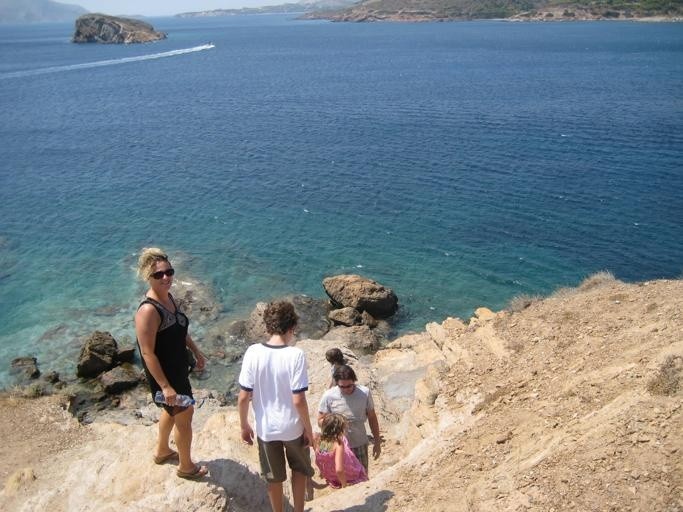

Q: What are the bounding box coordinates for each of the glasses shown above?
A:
[149,268,174,280]
[337,383,353,388]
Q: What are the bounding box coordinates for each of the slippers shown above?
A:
[177,464,208,480]
[153,450,179,464]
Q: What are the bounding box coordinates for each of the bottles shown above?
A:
[154,389,196,408]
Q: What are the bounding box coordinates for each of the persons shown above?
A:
[135,248,208,481]
[309,412,370,489]
[237,300,317,511]
[316,365,381,478]
[324,348,347,389]
